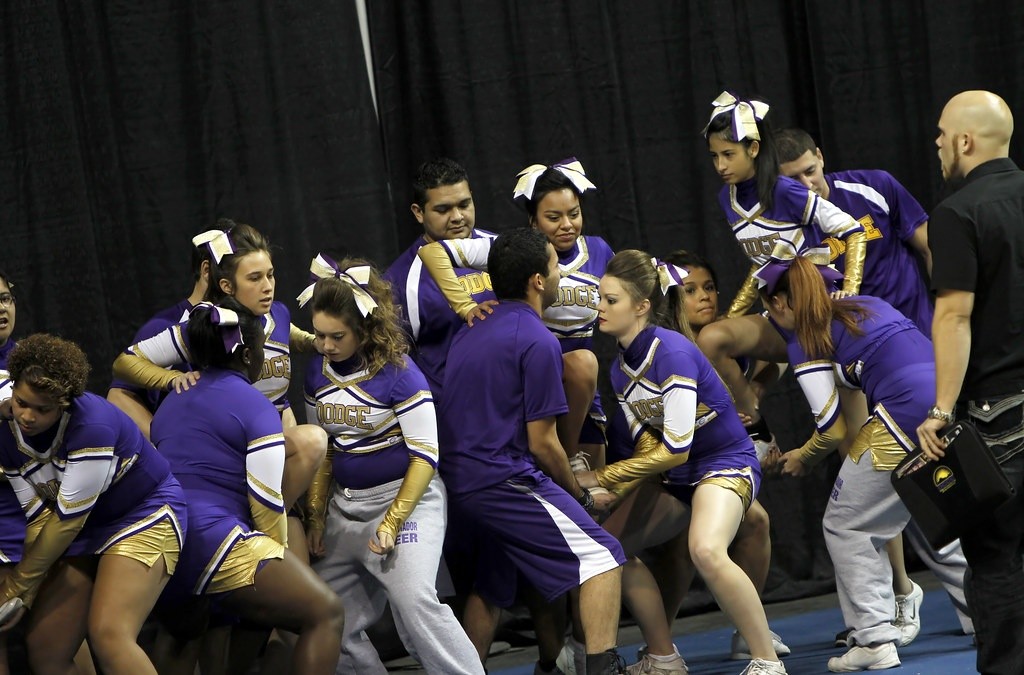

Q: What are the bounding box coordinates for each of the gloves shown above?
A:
[0,569,44,610]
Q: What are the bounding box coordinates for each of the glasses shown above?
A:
[0,293,15,307]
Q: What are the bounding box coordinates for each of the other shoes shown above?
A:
[533,660,566,675]
[585,649,628,675]
[0,597,27,631]
[555,645,578,675]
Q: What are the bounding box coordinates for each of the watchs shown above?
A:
[927,404,953,425]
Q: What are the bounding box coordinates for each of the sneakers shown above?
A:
[624,642,689,675]
[748,431,781,469]
[742,659,788,675]
[893,579,923,646]
[828,641,901,672]
[730,627,791,659]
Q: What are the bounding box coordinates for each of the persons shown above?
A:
[915,90,1024,675]
[0,93,978,675]
